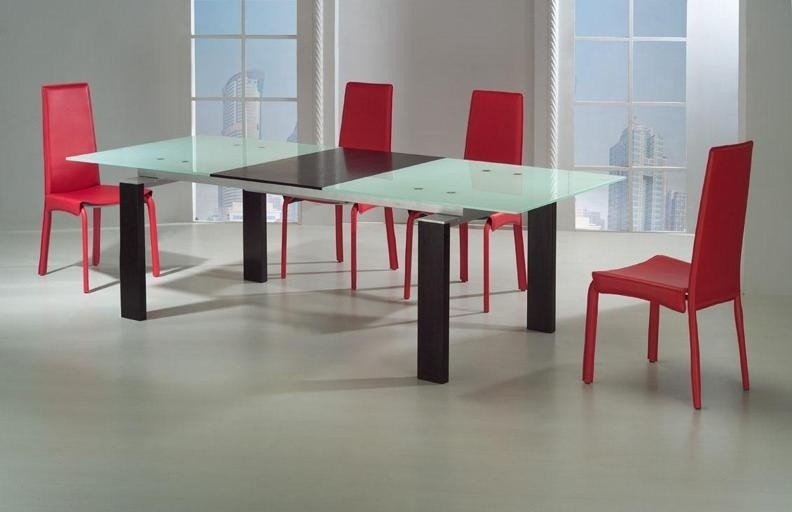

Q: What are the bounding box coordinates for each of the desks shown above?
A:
[65,134,626,384]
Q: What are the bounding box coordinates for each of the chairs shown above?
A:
[37,83,161,294]
[581,141,751,410]
